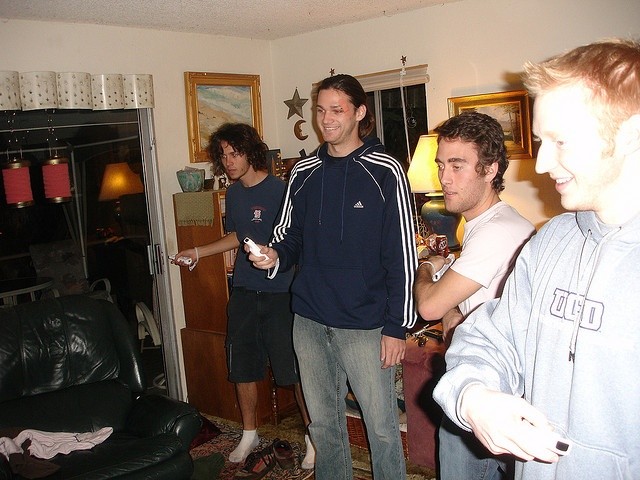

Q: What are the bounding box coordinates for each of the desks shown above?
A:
[0,274,58,306]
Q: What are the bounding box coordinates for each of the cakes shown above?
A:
[417,244,429,260]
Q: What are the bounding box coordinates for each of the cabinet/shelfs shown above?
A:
[176,328,298,427]
[174,191,239,333]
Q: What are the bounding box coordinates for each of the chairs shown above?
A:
[29,238,113,304]
[134,276,163,355]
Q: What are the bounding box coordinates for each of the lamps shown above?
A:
[42,112,72,205]
[98,159,144,234]
[0,111,36,210]
[406,134,462,249]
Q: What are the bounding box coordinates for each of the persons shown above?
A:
[412,111,537,479]
[244,75,420,480]
[171,123,317,469]
[431,40,640,479]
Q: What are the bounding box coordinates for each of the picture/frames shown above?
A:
[446,89,534,161]
[184,71,264,163]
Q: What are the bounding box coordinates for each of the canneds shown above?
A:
[437,236,448,257]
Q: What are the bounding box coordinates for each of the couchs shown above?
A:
[0,292,225,480]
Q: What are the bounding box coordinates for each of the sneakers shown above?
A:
[232,446,276,479]
[272,438,295,470]
